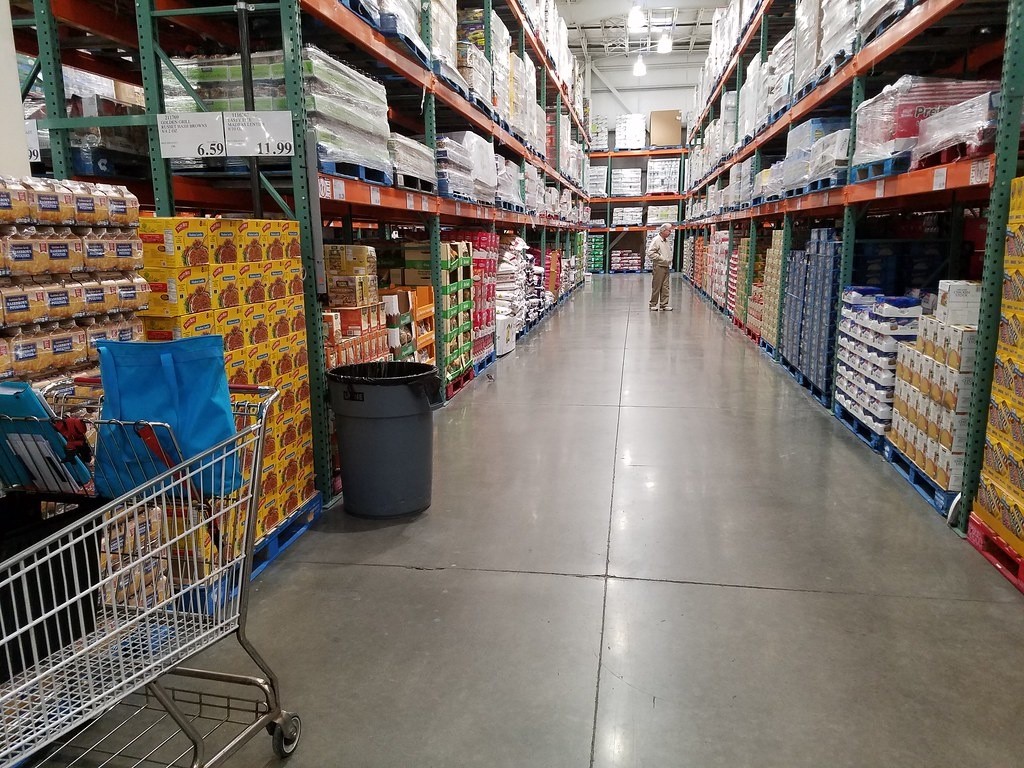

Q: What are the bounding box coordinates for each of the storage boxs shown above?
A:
[9,1,593,587]
[682,0,996,219]
[683,223,980,493]
[587,205,678,271]
[974,176,1024,558]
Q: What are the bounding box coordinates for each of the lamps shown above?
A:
[657,32,672,54]
[633,53,646,76]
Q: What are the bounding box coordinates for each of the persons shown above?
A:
[648,223,673,312]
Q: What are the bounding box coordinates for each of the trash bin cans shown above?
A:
[322,352,457,535]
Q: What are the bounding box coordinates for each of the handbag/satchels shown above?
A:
[96,334,246,502]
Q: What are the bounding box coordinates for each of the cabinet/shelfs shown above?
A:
[587,147,690,274]
[679,0,1024,594]
[0,0,594,648]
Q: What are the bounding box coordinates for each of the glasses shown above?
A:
[665,230,671,232]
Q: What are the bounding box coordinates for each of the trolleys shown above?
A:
[1,375,303,768]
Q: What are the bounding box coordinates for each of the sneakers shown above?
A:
[648,307,658,311]
[660,307,673,310]
